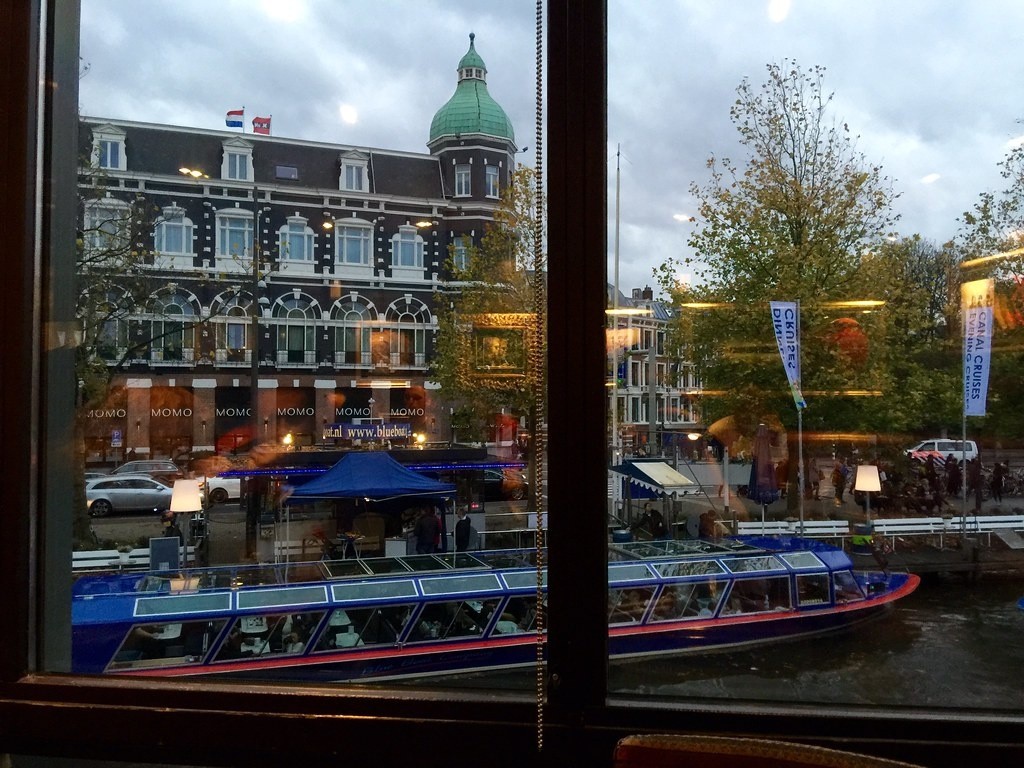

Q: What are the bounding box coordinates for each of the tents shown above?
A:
[284,452,457,571]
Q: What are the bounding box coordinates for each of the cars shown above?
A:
[84,471,172,519]
[193,472,240,505]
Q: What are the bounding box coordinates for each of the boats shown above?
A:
[72,534,922,686]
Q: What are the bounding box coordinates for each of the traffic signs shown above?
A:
[111,429,123,448]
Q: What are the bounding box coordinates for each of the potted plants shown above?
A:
[940,513,953,527]
[784,517,799,530]
[117,545,133,562]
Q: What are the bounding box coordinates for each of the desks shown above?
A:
[329,610,351,626]
[496,621,525,633]
[465,600,483,613]
[241,615,268,633]
[335,633,365,647]
[241,637,270,654]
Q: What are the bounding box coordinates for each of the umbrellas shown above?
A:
[746,423,779,537]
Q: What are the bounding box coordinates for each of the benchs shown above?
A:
[738,521,853,550]
[70,545,196,573]
[873,515,1024,547]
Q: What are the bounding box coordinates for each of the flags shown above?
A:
[252,117,270,134]
[226,110,243,128]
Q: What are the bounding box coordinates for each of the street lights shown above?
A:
[368,398,376,452]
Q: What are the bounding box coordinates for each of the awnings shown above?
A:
[611,461,700,495]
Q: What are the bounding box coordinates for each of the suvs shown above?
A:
[903,439,978,472]
[110,459,189,489]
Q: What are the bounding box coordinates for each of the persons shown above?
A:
[455,508,472,552]
[271,613,306,654]
[776,453,1010,503]
[626,502,664,541]
[132,624,163,637]
[219,626,255,660]
[412,502,442,554]
[698,510,716,538]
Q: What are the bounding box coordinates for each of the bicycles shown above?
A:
[939,463,1024,502]
